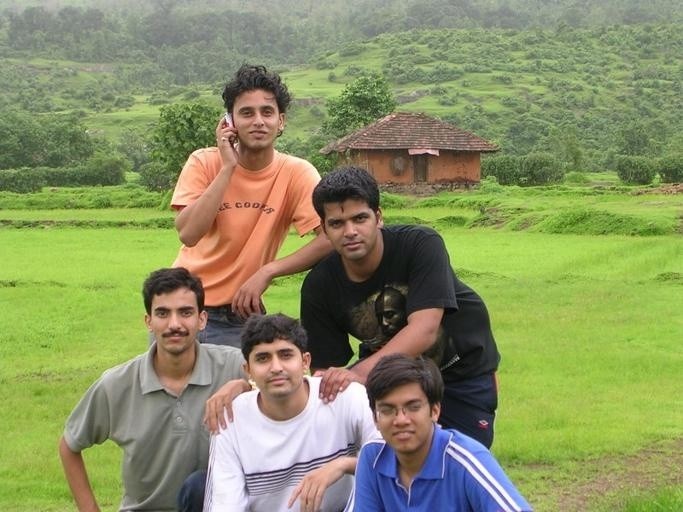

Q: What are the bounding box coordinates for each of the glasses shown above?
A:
[374,400,428,418]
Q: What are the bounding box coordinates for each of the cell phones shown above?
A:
[223,112,237,143]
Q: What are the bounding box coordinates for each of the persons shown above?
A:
[202,313,386,512]
[343,352,534,512]
[300,167,501,450]
[58,266,254,512]
[149,62,335,348]
[359,288,456,370]
